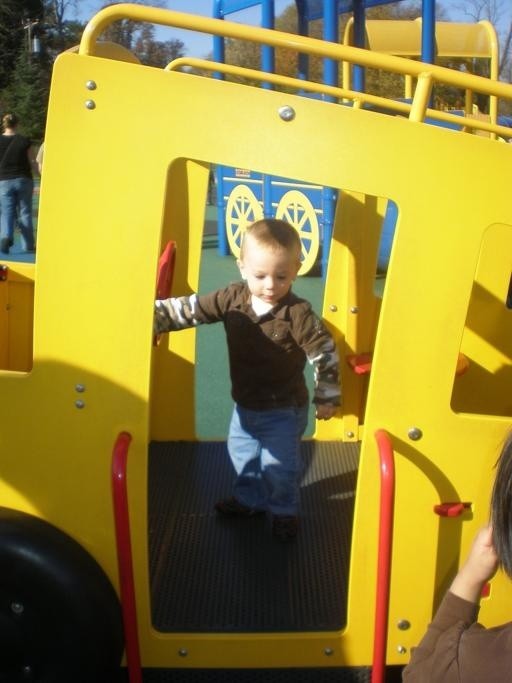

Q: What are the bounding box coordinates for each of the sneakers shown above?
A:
[269,514,300,544]
[213,498,266,517]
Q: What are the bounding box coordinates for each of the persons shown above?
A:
[36,142,45,173]
[155,218,343,543]
[396,429,510,683]
[1,112,36,252]
